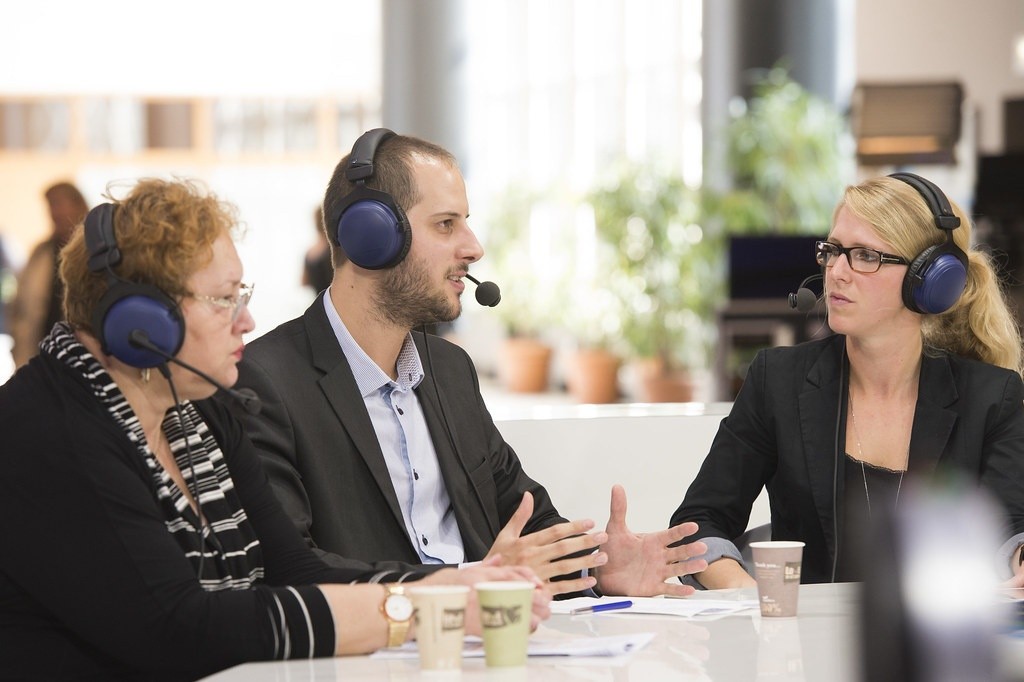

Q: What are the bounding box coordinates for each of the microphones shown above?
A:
[787,274,823,313]
[465,274,502,307]
[156,350,263,418]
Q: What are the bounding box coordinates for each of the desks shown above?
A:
[202,578,866,682]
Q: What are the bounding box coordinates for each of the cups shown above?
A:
[411,585,471,678]
[473,580,536,667]
[748,540,806,618]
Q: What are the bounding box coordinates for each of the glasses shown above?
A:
[814,241,911,273]
[182,284,254,323]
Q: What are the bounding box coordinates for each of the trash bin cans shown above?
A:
[570,601,633,615]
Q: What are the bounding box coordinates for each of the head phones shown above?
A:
[886,172,971,314]
[82,202,187,379]
[334,128,413,271]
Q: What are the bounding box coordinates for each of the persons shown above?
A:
[9,183,91,370]
[663,172,1024,682]
[230,127,710,599]
[0,176,548,682]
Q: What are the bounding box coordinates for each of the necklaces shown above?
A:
[848,389,910,520]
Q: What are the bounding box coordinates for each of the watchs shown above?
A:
[378,580,415,648]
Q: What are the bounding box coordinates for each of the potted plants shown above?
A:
[492,169,721,403]
